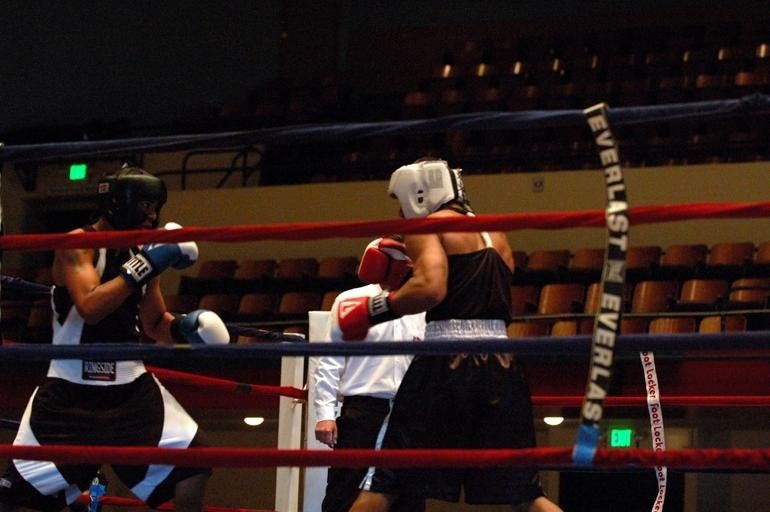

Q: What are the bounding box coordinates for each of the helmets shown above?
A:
[94,162,167,230]
[388,160,470,219]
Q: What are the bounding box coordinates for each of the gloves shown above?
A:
[119,221,200,287]
[170,310,231,347]
[326,282,402,342]
[357,238,412,292]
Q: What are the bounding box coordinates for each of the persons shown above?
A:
[313,235,430,512]
[328,156,566,510]
[0,167,230,512]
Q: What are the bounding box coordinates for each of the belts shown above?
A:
[340,395,400,413]
